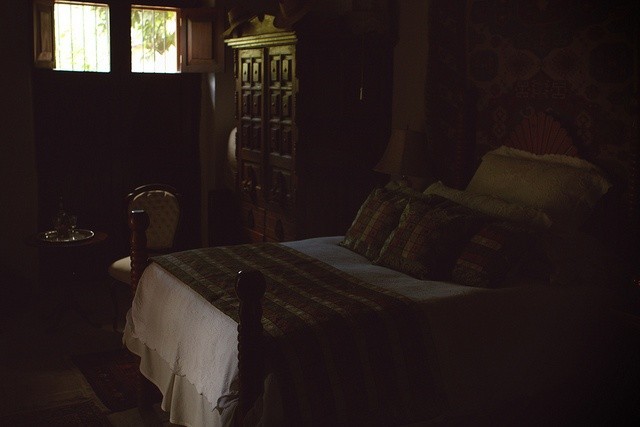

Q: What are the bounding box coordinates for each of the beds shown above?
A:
[120,208,585,426]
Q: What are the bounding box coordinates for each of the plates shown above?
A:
[38,229,95,243]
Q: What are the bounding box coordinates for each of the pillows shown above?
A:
[422,180,550,229]
[374,194,529,289]
[464,146,610,221]
[336,185,407,265]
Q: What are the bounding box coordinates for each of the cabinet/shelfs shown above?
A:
[224,31,310,243]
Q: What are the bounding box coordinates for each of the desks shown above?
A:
[25,224,110,336]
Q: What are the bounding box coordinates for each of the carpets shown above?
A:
[64,347,142,416]
[0,399,116,427]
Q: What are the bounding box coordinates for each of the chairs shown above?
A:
[108,182,185,336]
